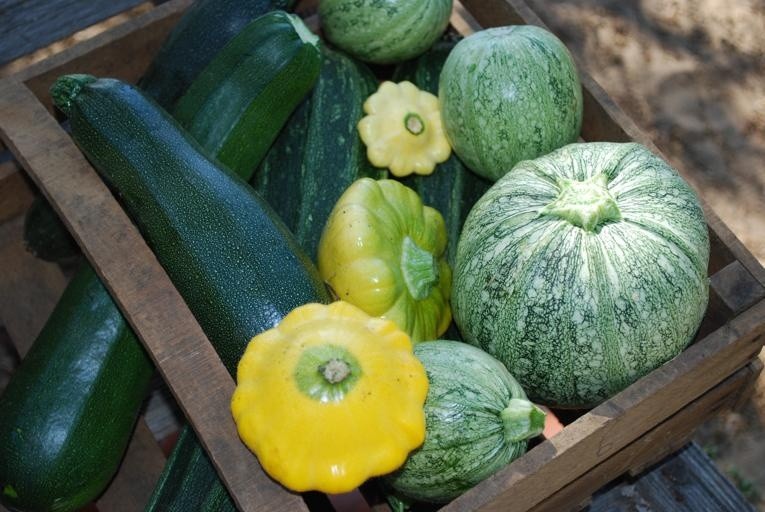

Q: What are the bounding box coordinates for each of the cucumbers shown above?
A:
[0,0,494,512]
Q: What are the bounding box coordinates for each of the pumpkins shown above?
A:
[227,0,712,498]
